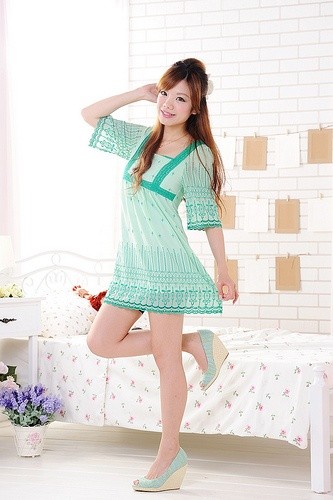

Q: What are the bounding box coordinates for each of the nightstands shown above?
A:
[0,292,40,382]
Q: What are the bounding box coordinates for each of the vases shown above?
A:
[1,428,54,467]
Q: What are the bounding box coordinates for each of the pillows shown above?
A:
[40,284,150,337]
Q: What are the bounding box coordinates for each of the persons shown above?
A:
[81,57,239,492]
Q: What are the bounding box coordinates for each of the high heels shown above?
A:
[133,447,187,491]
[197,329,228,391]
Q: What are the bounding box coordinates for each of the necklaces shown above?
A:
[158,132,188,148]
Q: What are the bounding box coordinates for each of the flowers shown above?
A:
[1,383,64,426]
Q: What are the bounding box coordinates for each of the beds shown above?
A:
[1,250,333,489]
